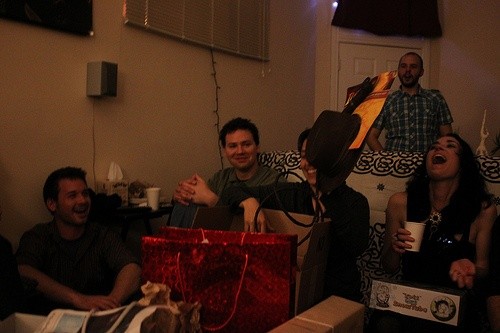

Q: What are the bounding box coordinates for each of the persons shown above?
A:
[166,115,287,230]
[219,126,371,303]
[368,49,454,151]
[368,131,500,332]
[14,165,144,313]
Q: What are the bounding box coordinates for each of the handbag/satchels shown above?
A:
[255,171,333,315]
[138,226,298,333]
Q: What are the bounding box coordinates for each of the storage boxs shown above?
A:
[191,207,331,315]
[266,294,365,333]
[369,281,467,326]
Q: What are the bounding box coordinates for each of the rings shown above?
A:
[390,232,400,241]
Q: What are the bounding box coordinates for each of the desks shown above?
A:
[98,204,171,240]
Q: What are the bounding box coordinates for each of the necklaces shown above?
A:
[429,206,444,241]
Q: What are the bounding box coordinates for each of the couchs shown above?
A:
[251,152,500,322]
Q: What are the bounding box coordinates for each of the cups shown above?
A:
[404,221,425,252]
[146,188,161,212]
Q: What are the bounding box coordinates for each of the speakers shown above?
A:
[86,60,118,98]
